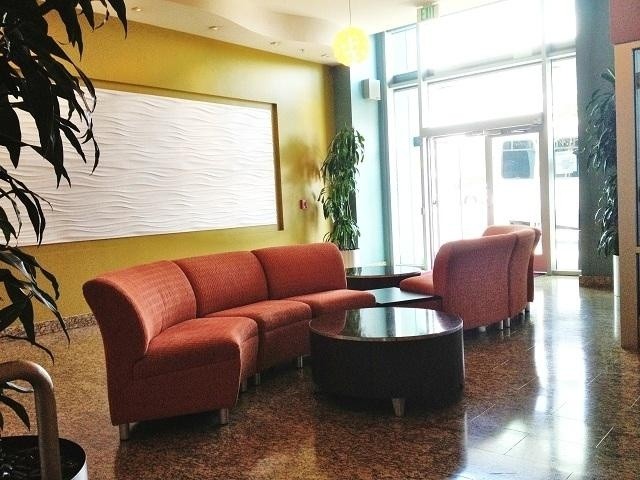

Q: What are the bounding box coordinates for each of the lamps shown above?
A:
[332,0,369,68]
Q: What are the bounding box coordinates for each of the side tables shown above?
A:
[366,286,442,311]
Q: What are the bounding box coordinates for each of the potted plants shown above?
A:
[316,118,366,268]
[0,0,127,480]
[583,67,621,296]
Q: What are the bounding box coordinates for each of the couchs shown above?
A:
[82,260,259,441]
[251,243,375,318]
[172,251,313,387]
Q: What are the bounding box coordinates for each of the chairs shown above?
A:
[400,224,540,332]
